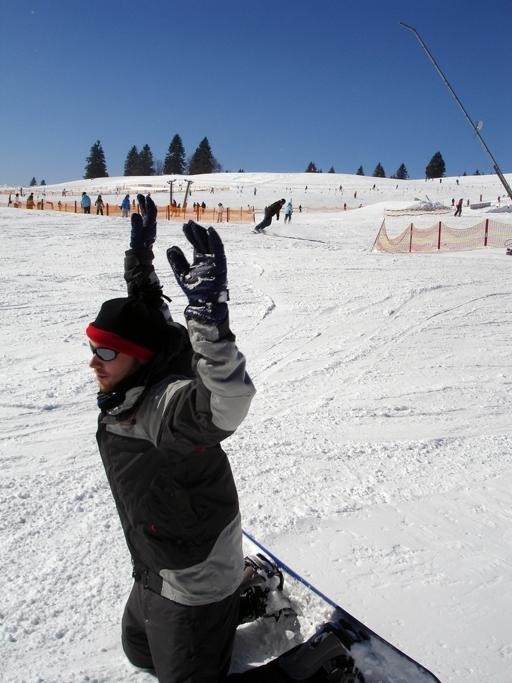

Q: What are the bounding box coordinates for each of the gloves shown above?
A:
[130,194,158,254]
[166,220,227,307]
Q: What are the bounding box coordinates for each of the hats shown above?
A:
[86,299,173,357]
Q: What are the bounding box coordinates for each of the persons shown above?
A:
[172,200,206,215]
[95,194,104,215]
[121,193,131,217]
[81,191,91,214]
[217,202,224,223]
[7,188,68,209]
[254,199,286,233]
[453,198,463,216]
[86,190,363,683]
[284,203,293,222]
[339,178,501,210]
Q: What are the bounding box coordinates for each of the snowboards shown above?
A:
[242,531,440,683]
[251,229,266,234]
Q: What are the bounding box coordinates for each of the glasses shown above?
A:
[87,338,118,361]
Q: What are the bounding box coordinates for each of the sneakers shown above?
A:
[244,553,298,624]
[308,616,372,683]
